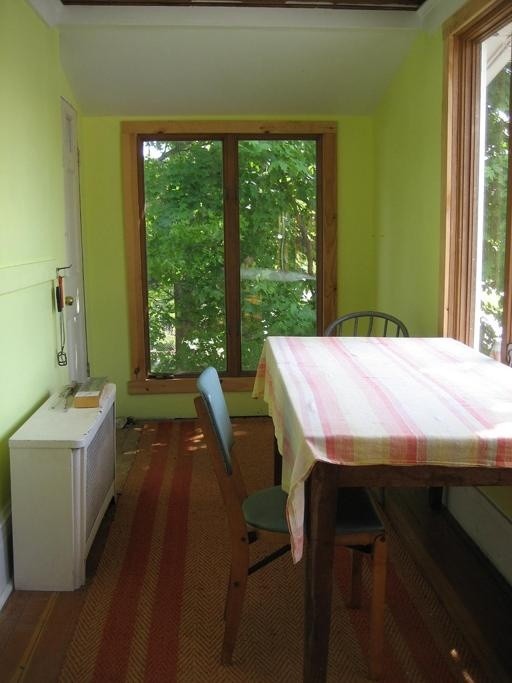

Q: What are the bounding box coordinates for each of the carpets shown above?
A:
[57,416,484,682]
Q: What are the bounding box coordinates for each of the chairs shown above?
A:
[194,367,388,683]
[323,310,409,337]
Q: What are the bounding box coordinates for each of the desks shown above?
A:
[8,382,119,592]
[251,335,512,682]
